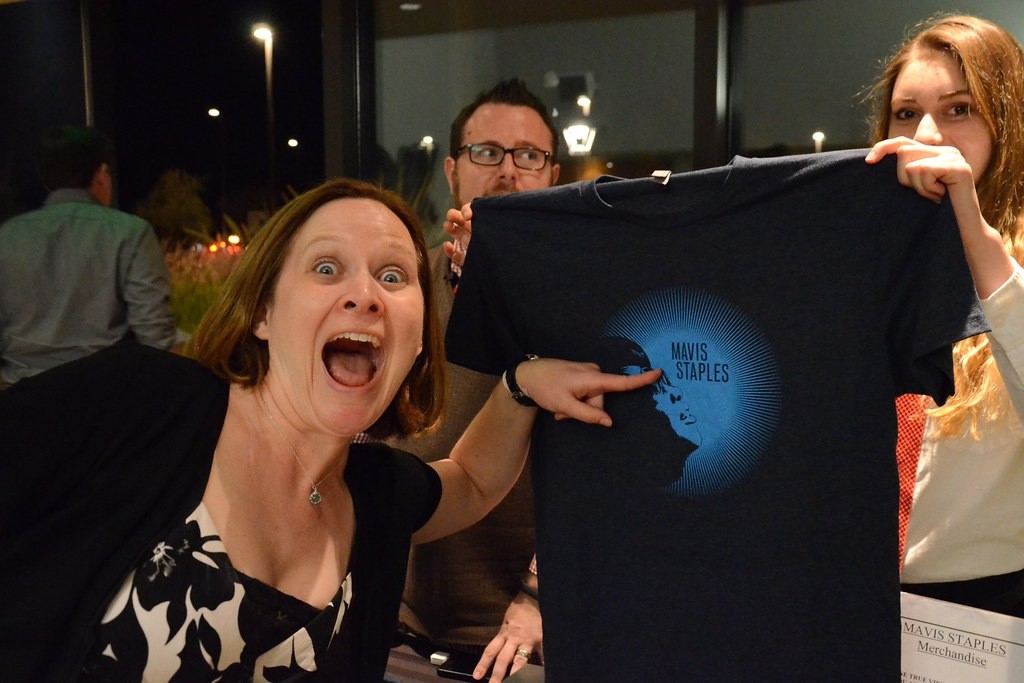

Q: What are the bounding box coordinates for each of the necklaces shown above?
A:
[259,389,342,504]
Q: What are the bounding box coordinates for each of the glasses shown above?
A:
[454,142,556,171]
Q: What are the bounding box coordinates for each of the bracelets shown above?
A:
[502,353,539,408]
[517,577,539,600]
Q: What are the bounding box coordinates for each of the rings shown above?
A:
[517,649,531,658]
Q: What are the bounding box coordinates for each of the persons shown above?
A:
[0,176,661,683]
[439,14,1024,615]
[381,75,544,683]
[0,125,181,391]
